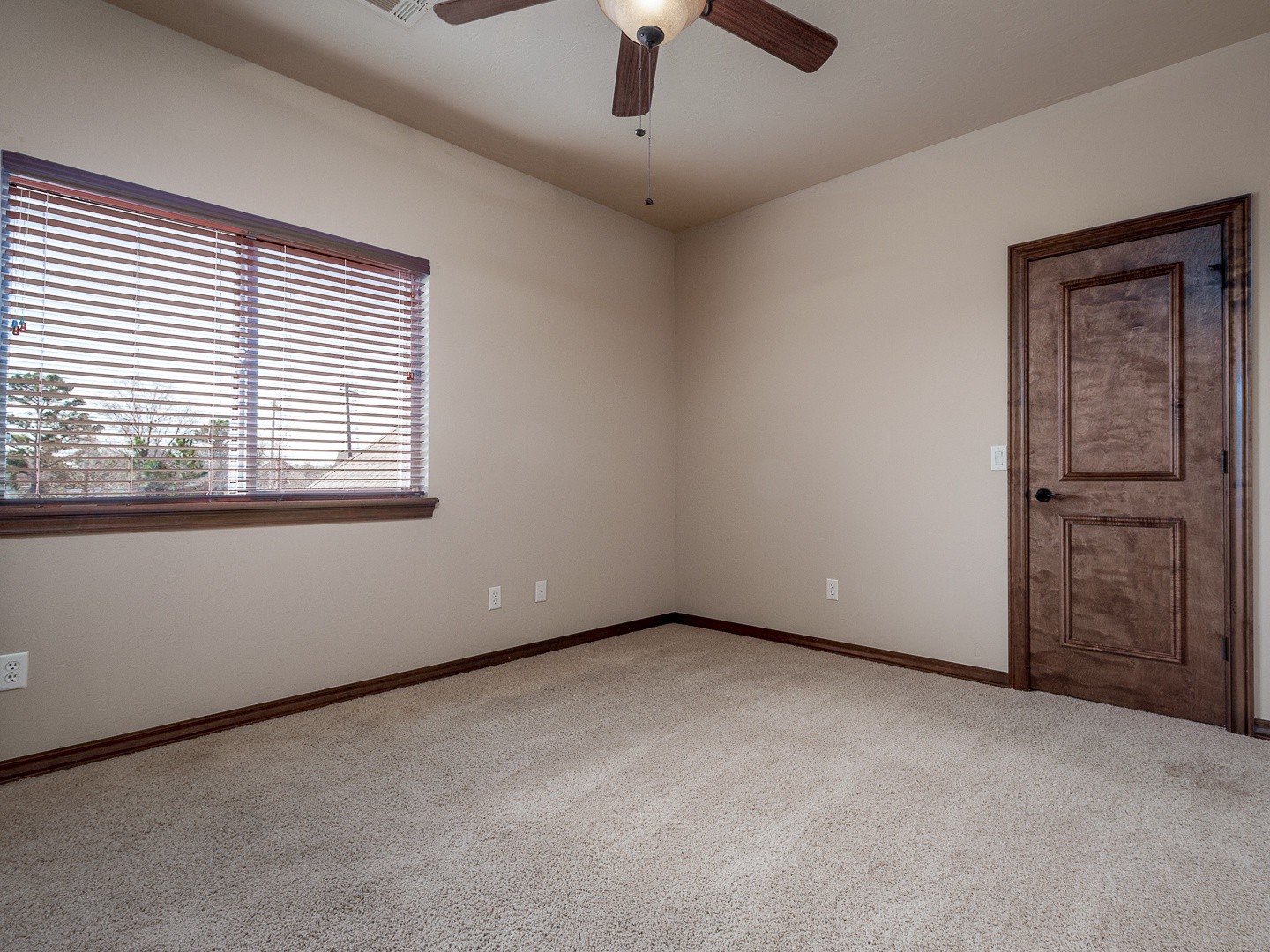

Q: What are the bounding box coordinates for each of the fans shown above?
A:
[432,0,837,116]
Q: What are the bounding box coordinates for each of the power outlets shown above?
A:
[825,579,838,600]
[0,650,29,691]
[488,587,501,610]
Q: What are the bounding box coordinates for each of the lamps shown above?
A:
[600,1,708,206]
